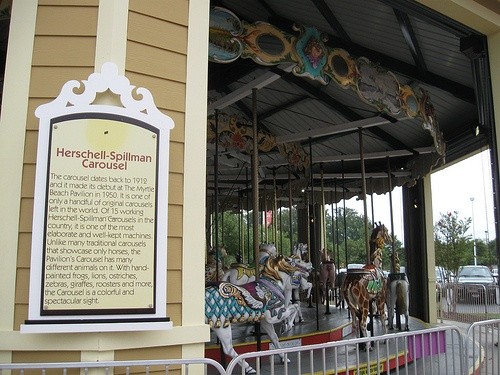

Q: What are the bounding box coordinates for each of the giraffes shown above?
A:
[343,221,392,352]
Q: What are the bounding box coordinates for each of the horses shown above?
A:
[205,243,227,281]
[204,255,301,374]
[384,248,410,332]
[222,242,277,285]
[284,242,314,327]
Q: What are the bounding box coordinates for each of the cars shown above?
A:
[434,265,499,305]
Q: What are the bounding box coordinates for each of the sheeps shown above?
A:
[319,248,336,291]
